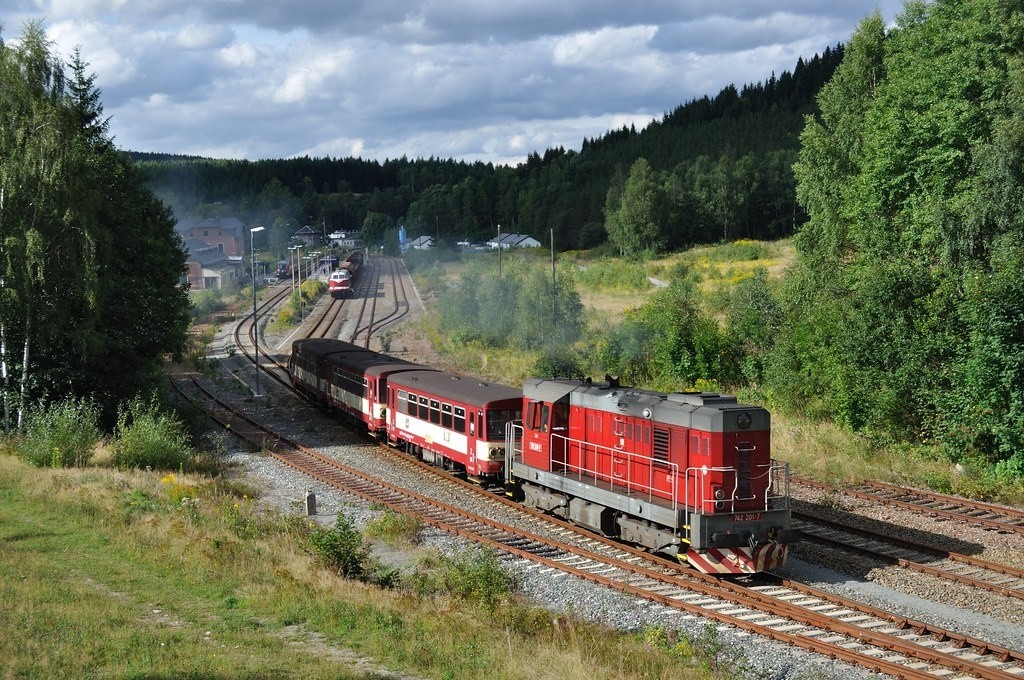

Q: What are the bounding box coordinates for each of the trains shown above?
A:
[287,338,802,575]
[328,249,366,299]
[275,253,298,279]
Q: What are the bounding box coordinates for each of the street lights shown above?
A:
[251,226,265,396]
